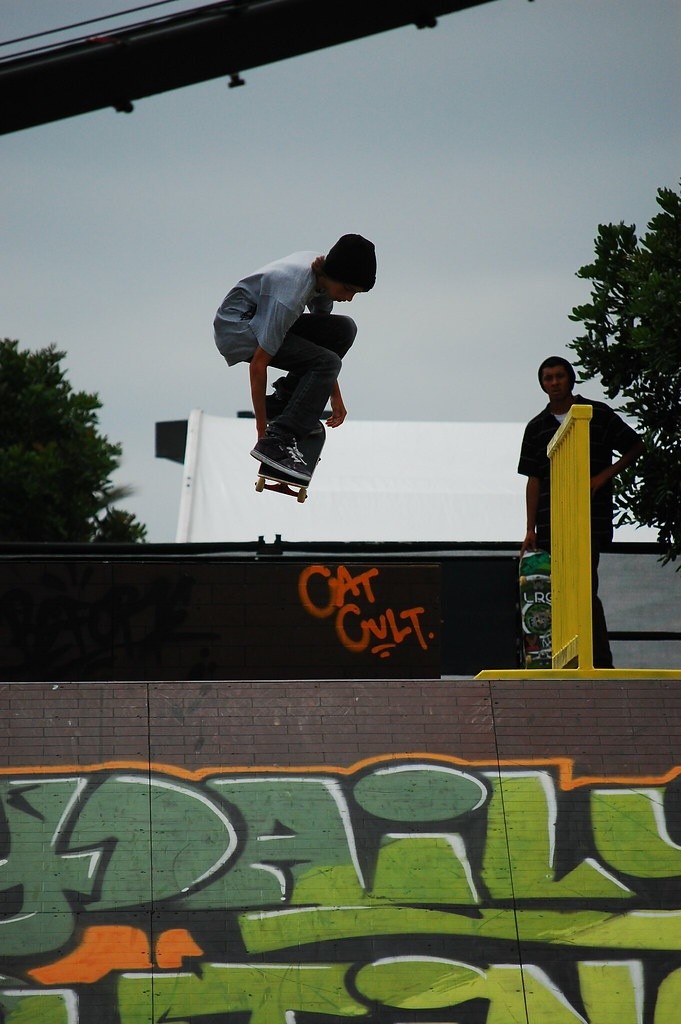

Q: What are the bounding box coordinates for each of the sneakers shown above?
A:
[265,396,323,435]
[251,436,311,482]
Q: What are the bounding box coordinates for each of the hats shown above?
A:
[323,234,377,290]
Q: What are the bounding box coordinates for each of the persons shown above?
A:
[213,234,377,480]
[518,356,646,669]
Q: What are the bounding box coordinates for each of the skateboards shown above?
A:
[518,548,551,668]
[254,417,326,503]
